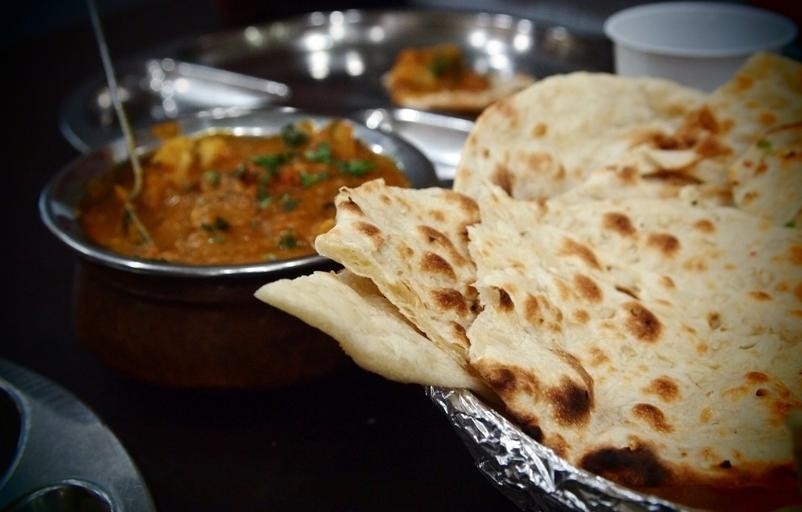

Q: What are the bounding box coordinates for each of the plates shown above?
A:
[39,110,437,303]
[194,5,605,120]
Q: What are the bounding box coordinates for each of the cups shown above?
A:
[604,4,797,94]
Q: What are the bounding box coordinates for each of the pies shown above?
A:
[254,50,802,512]
